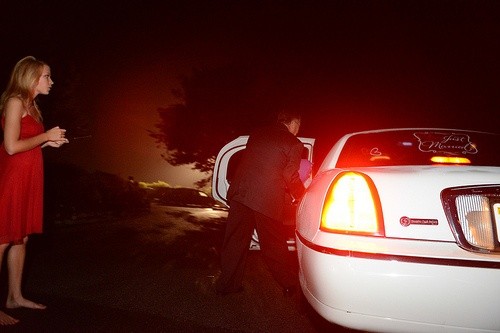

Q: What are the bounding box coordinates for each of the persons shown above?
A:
[211,107,305,297]
[0,55,69,328]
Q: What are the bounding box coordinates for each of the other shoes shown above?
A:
[284,285,297,297]
[216,286,244,297]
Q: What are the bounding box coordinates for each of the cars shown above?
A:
[212,127,500,333]
